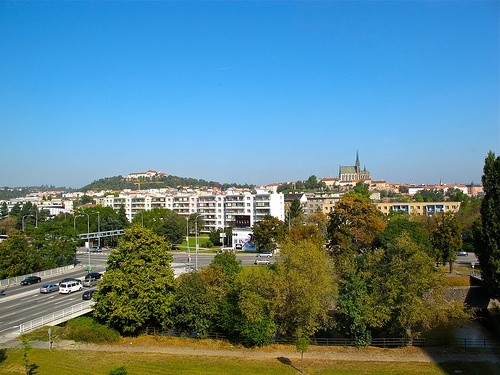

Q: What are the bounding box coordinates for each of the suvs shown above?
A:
[256,252,273,258]
[90,246,102,252]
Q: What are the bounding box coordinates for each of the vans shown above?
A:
[58,281,83,294]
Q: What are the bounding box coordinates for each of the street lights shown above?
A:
[73,214,84,230]
[97,211,100,246]
[78,211,99,274]
[22,214,33,232]
[195,213,206,272]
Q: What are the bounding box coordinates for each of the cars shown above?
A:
[82,290,98,300]
[59,278,82,285]
[457,249,468,257]
[255,258,271,265]
[20,276,41,286]
[85,272,103,279]
[83,278,100,288]
[40,284,59,293]
